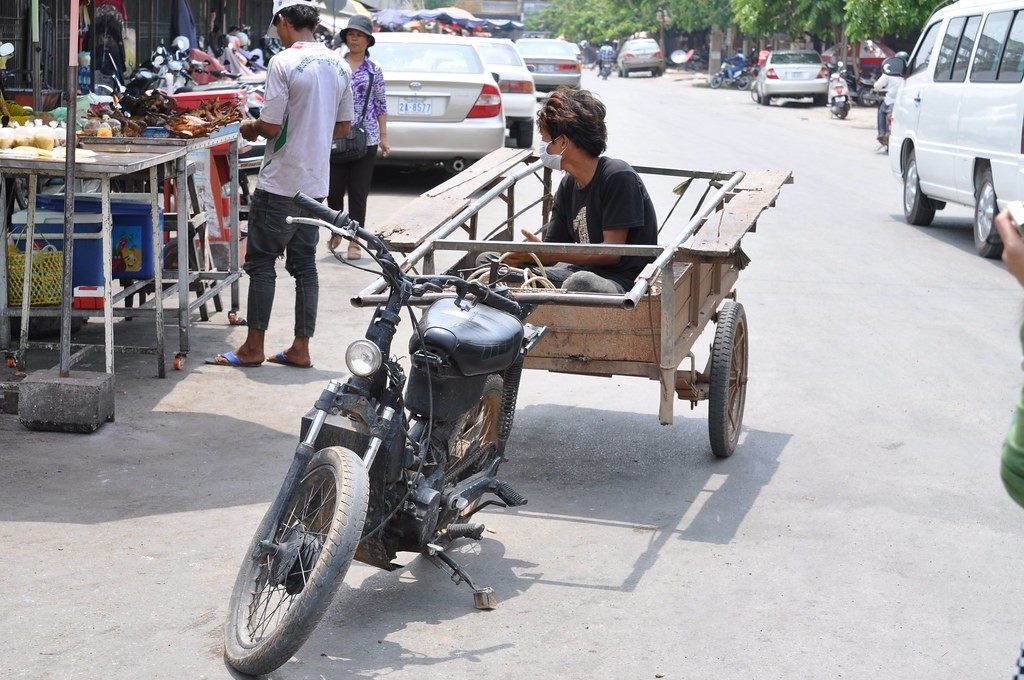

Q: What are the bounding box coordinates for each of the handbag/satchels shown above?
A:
[329,122,366,163]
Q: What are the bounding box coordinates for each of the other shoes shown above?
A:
[333,236,341,245]
[347,244,362,259]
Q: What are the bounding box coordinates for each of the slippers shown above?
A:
[266,352,313,368]
[205,350,261,367]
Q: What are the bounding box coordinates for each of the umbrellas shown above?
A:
[819,39,897,65]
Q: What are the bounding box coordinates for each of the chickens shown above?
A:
[82,91,242,141]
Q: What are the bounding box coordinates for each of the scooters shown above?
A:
[111,25,259,122]
[225,192,548,674]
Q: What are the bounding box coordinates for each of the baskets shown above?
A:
[6,224,62,306]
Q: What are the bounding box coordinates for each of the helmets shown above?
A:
[171,36,189,52]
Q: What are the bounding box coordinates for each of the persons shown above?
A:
[994,208,1024,680]
[471,86,657,294]
[202,1,357,369]
[872,50,909,136]
[226,24,252,65]
[326,15,392,261]
[597,38,615,74]
[725,48,747,82]
[759,45,775,69]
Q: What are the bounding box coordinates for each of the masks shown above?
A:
[539,136,570,172]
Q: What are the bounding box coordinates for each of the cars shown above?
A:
[752,48,833,108]
[620,38,664,77]
[332,32,536,182]
[514,37,581,101]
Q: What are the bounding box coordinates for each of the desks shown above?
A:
[5,89,62,113]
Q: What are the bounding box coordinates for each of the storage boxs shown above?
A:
[35,193,165,281]
[10,209,104,296]
[75,287,105,310]
[168,90,247,150]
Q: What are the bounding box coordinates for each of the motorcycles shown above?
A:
[870,88,896,153]
[827,63,853,118]
[710,59,750,90]
[847,75,886,106]
[594,61,616,80]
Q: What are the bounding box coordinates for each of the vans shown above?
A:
[888,0,1024,259]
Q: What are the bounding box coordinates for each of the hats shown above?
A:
[340,14,375,47]
[266,0,317,38]
